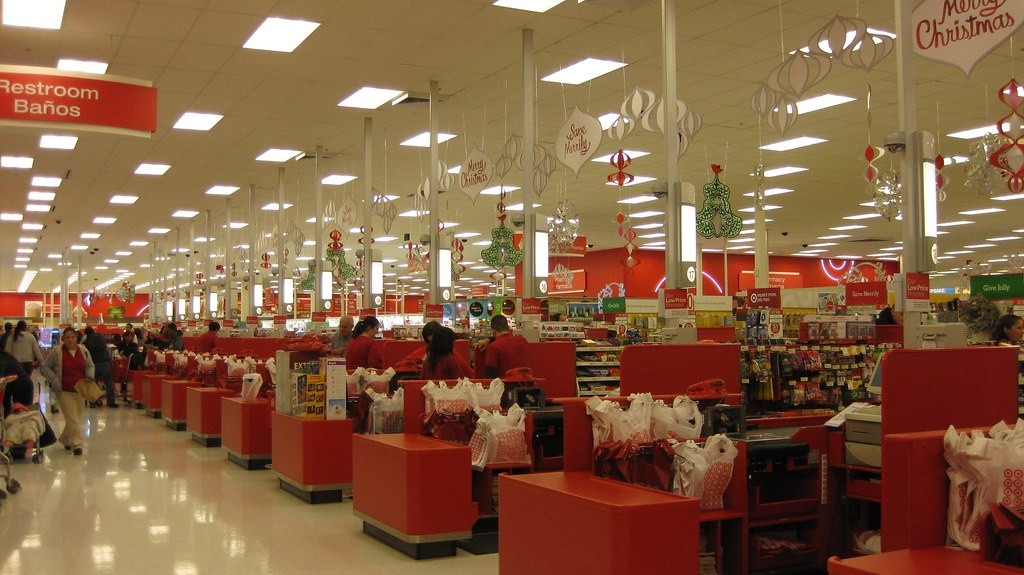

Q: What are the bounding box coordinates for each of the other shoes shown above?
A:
[109,403,120,409]
[74,448,82,456]
[58,438,71,450]
[88,402,103,408]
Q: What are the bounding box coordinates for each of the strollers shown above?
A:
[0,375,22,506]
[7,381,45,464]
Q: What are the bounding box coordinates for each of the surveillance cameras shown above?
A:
[309,260,315,268]
[90,247,100,255]
[256,273,259,275]
[882,130,908,154]
[95,279,98,280]
[272,269,278,276]
[193,251,198,254]
[355,249,363,259]
[186,254,190,257]
[243,276,250,282]
[510,212,525,227]
[782,232,788,236]
[220,281,226,286]
[588,245,593,248]
[802,244,808,248]
[56,220,61,224]
[391,265,394,267]
[652,181,667,198]
[461,239,467,242]
[419,234,430,245]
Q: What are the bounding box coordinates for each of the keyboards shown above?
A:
[850,405,882,416]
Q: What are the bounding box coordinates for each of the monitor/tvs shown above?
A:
[868,352,887,394]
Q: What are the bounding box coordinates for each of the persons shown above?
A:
[552,313,566,321]
[30,327,39,343]
[476,314,529,378]
[198,321,221,353]
[41,326,96,455]
[45,379,59,414]
[853,305,903,362]
[331,315,354,355]
[420,327,465,379]
[78,327,119,408]
[5,320,43,376]
[406,321,475,379]
[345,315,382,369]
[114,322,184,369]
[0,323,13,353]
[993,314,1024,345]
[3,402,45,456]
[0,352,34,406]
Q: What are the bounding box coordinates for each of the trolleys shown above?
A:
[94,353,134,406]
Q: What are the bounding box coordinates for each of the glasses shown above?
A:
[338,324,353,329]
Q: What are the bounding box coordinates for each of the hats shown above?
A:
[149,328,159,336]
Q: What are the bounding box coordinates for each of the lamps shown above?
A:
[165,230,454,323]
[676,200,697,290]
[531,213,549,299]
[872,162,902,222]
[653,179,667,198]
[912,129,940,273]
[546,167,581,254]
[883,129,906,152]
[511,211,526,227]
[962,77,1011,198]
